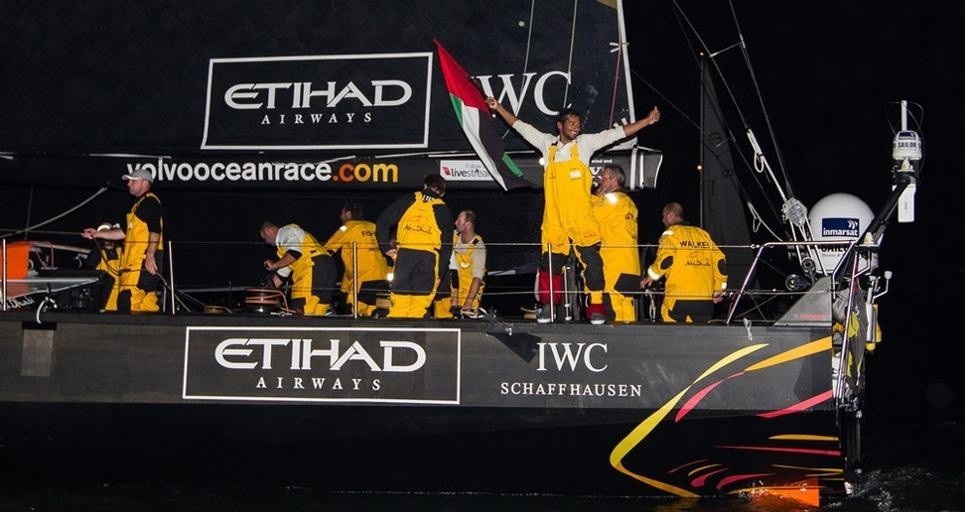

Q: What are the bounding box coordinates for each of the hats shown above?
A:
[121,169,154,183]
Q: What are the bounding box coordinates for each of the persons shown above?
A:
[484,96,663,327]
[374,174,455,321]
[259,217,339,318]
[640,202,730,325]
[589,163,643,323]
[447,211,489,319]
[79,168,168,314]
[319,200,394,320]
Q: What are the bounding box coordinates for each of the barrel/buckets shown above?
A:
[245,287,281,315]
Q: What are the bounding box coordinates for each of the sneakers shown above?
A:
[590,312,605,325]
[522,302,544,311]
[538,304,557,323]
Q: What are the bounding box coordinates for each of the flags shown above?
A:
[433,34,527,194]
